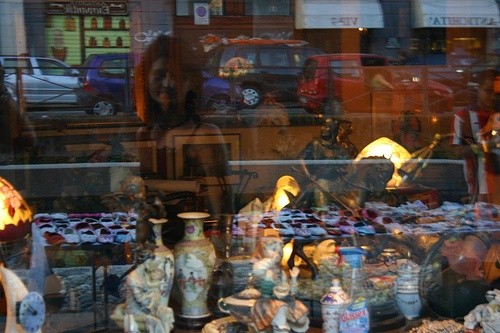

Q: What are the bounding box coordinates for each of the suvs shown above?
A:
[3,54,79,108]
[76,52,246,117]
[206,44,326,108]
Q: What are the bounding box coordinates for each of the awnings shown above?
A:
[295,1,384,32]
[410,1,500,32]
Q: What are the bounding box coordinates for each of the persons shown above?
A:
[450,71,500,208]
[0,65,38,191]
[370,69,395,90]
[337,119,357,161]
[122,35,230,248]
[393,109,422,148]
[298,116,351,223]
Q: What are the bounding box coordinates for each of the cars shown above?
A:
[297,51,500,116]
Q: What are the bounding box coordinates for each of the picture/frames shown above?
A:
[64,137,158,175]
[360,0,447,66]
[173,133,242,186]
